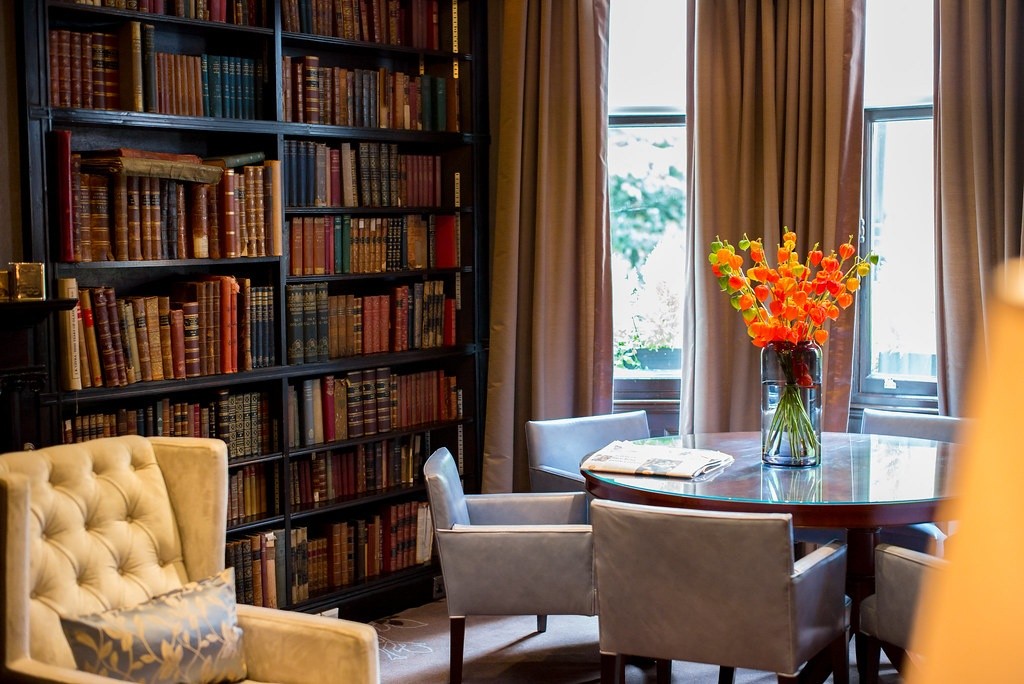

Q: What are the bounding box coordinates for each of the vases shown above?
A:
[759,340,823,470]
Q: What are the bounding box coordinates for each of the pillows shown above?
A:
[58,567,248,684]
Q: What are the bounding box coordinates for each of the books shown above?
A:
[48,0,286,611]
[282,0,466,605]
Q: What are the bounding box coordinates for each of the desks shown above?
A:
[579,432,960,684]
[0,299,78,453]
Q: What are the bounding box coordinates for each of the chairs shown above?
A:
[857,533,956,684]
[589,497,852,684]
[527,409,651,633]
[423,447,598,684]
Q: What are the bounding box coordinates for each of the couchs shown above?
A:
[0,437,381,684]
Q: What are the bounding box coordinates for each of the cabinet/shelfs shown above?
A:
[21,0,490,626]
